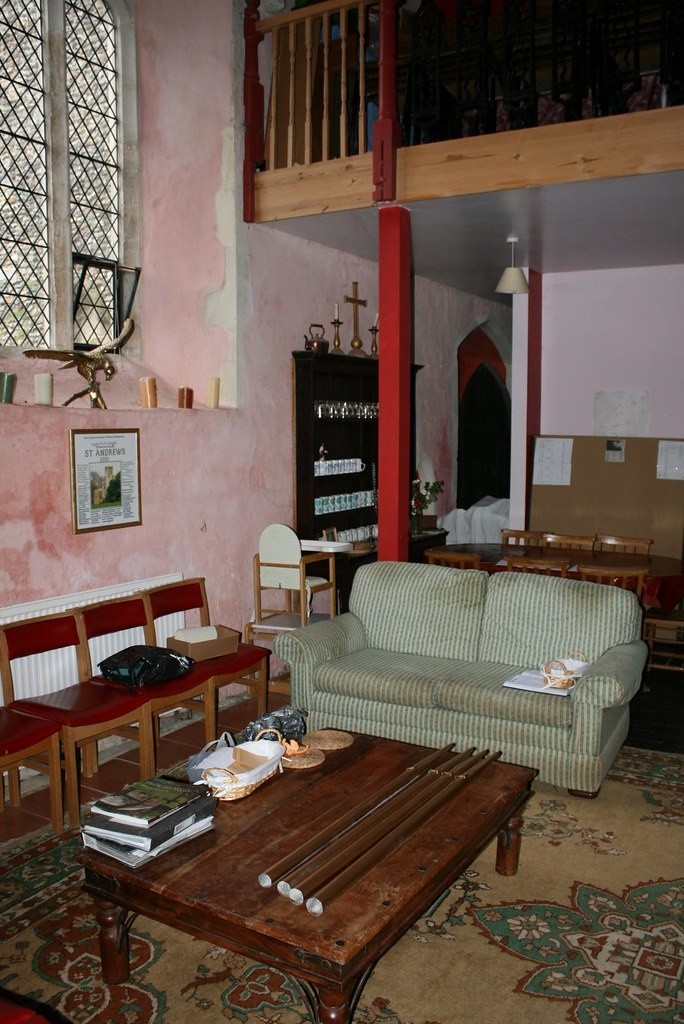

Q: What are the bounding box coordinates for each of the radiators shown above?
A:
[0,571,187,778]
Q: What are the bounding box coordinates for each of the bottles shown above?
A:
[314,400,379,418]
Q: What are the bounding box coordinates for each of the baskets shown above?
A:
[202,728,282,800]
[543,652,586,688]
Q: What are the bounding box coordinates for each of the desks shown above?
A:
[427,542,683,611]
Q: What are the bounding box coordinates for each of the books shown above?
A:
[80,777,218,868]
[501,669,571,697]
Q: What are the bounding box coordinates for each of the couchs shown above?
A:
[272,562,648,798]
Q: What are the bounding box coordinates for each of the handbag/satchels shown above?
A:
[98,645,195,692]
[188,731,234,783]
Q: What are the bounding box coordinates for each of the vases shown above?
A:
[410,509,422,536]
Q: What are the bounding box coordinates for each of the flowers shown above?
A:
[410,478,444,530]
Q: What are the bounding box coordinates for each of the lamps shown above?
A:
[494,237,531,293]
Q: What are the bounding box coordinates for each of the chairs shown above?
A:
[353,0,684,155]
[0,578,271,836]
[423,527,684,672]
[247,524,335,694]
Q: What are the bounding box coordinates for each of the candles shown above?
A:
[0,372,16,403]
[177,386,194,408]
[139,377,157,408]
[334,302,380,326]
[207,375,221,408]
[35,373,53,405]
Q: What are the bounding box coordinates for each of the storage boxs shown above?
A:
[167,624,239,660]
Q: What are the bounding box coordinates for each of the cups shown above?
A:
[139,377,157,409]
[337,525,378,542]
[34,373,53,406]
[314,490,375,515]
[0,372,17,403]
[178,386,194,408]
[314,458,366,476]
[206,378,220,409]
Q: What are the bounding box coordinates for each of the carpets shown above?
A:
[1,746,684,1024]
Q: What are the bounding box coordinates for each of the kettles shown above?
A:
[304,323,329,352]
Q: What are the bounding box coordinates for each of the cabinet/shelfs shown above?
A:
[292,350,450,615]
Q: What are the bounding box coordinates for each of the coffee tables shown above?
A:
[74,727,539,1024]
